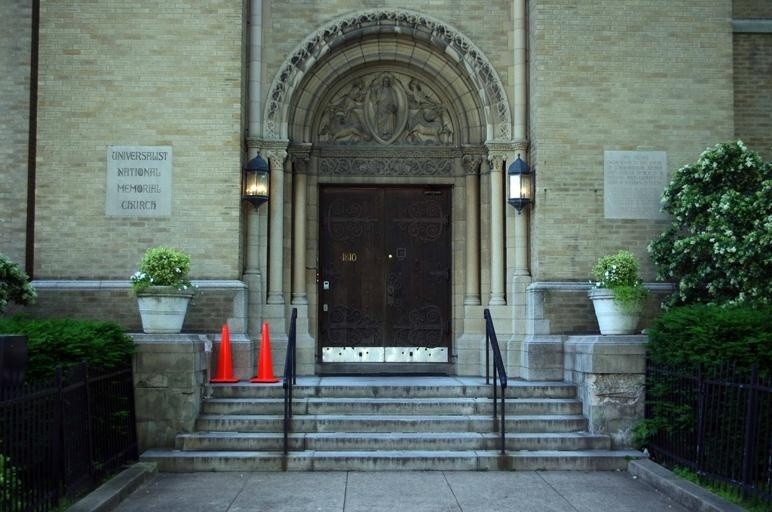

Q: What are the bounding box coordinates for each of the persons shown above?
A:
[321,73,451,146]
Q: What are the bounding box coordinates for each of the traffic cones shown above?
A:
[249,322,281,383]
[210,323,240,382]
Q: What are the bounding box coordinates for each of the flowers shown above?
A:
[127,246,199,292]
[588,245,650,314]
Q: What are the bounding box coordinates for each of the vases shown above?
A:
[590,287,644,336]
[132,285,196,336]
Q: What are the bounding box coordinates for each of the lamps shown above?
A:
[241,150,273,215]
[506,154,539,218]
